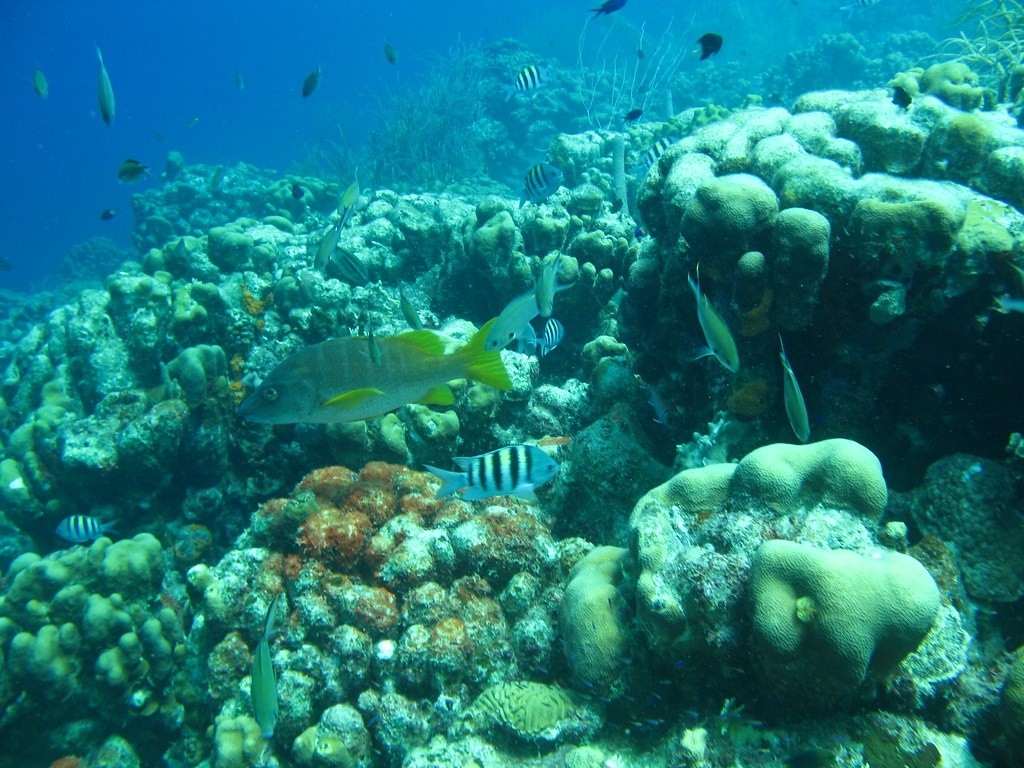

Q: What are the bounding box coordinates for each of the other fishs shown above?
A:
[2,1,1024,768]
